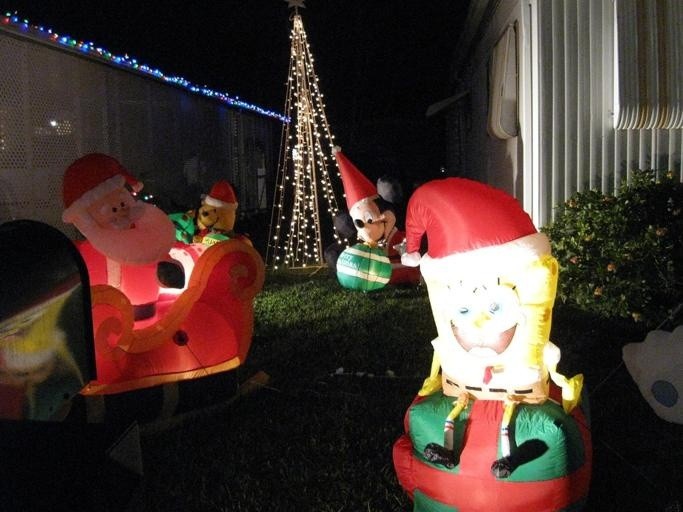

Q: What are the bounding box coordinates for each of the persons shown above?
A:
[60,151,266,398]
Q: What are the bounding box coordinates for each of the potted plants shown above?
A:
[552,168,683,429]
